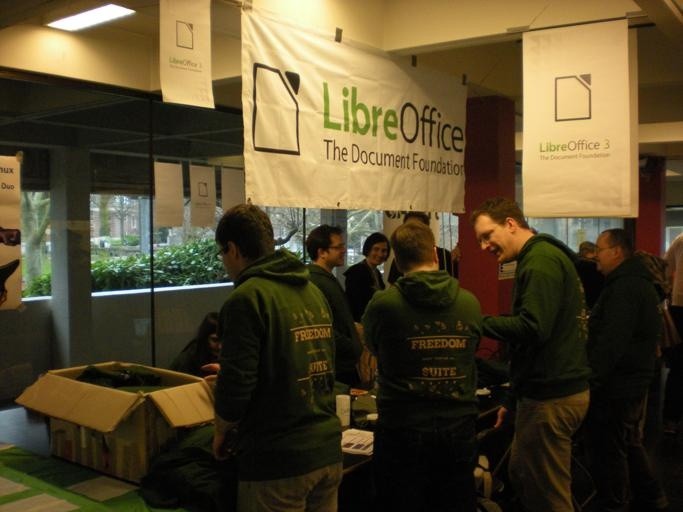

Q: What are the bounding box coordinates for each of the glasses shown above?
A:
[212,245,232,260]
[475,221,503,244]
[318,244,347,253]
[592,241,626,255]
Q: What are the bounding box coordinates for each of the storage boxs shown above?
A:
[14,358,218,482]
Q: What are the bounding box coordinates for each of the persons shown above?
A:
[307,223,360,391]
[390,212,458,287]
[589,229,666,511]
[203,204,343,511]
[344,231,389,386]
[470,196,589,511]
[663,230,683,430]
[362,224,482,511]
[572,241,599,281]
